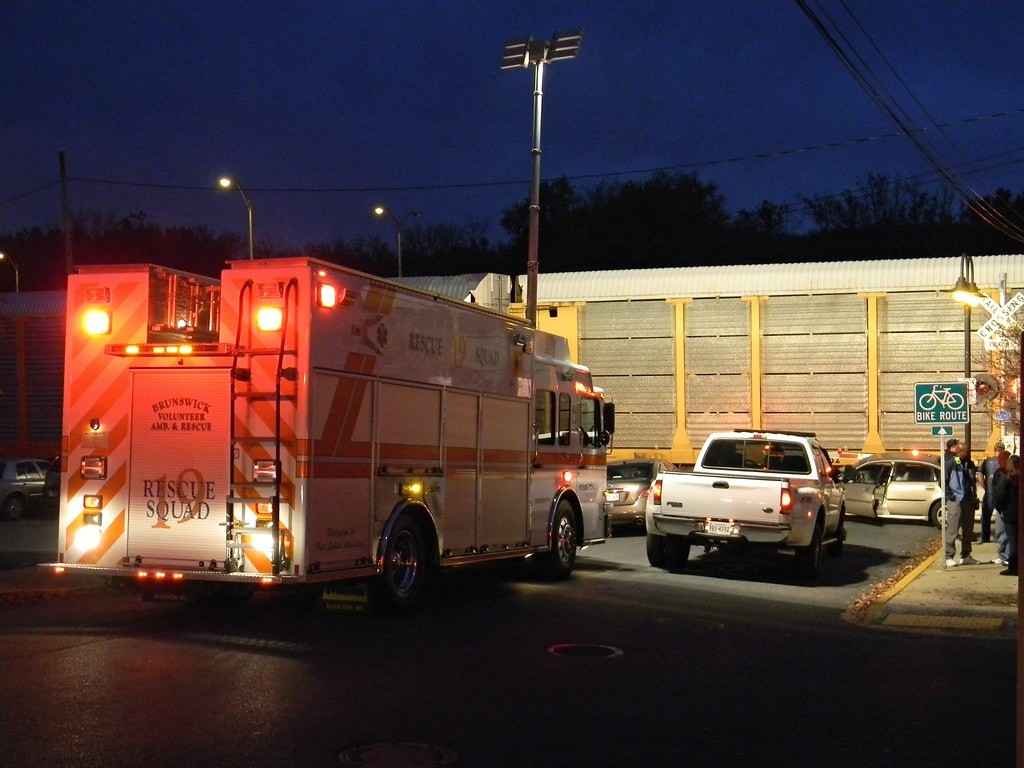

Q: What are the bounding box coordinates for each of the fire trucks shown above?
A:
[39,256,616,604]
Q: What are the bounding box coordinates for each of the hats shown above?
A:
[946,438,959,447]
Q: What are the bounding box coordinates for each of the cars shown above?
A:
[606,458,682,534]
[839,453,986,531]
[0,457,52,522]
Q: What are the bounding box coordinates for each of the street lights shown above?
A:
[220,171,282,260]
[375,199,423,276]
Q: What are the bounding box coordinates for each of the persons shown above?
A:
[999,455,1019,577]
[937,443,981,566]
[991,451,1011,564]
[976,443,1005,542]
[944,439,958,460]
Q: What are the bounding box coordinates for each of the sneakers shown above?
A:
[1002,561,1009,566]
[990,558,1002,564]
[946,559,957,566]
[960,556,980,565]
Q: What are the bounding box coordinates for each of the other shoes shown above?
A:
[1000,569,1018,576]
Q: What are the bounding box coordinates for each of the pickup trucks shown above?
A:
[645,427,846,573]
